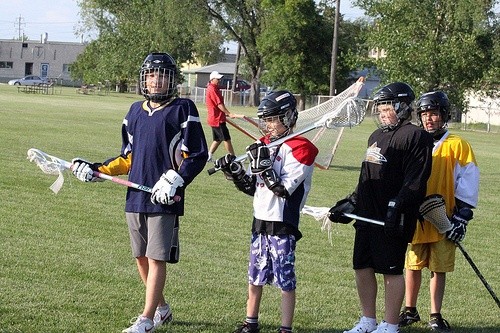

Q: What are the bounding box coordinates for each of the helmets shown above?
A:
[138,53,178,102]
[416,92,452,127]
[256,89,299,128]
[373,82,414,116]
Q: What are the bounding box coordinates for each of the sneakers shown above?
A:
[233,316,259,333]
[342,315,378,333]
[121,315,154,333]
[370,320,400,333]
[153,303,172,327]
[430,313,451,331]
[399,307,421,327]
[277,326,293,333]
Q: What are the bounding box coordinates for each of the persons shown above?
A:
[206,71,235,161]
[215,89,319,333]
[72,52,208,333]
[327,82,434,333]
[399,89,480,333]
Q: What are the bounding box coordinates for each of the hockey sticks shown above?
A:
[27,148,182,201]
[300,204,385,225]
[419,193,498,302]
[208,97,366,175]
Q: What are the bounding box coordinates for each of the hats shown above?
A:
[209,71,224,81]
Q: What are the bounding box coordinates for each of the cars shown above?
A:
[8,75,50,87]
[218,78,251,91]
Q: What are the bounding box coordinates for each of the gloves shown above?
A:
[328,192,358,223]
[150,169,185,206]
[445,206,474,243]
[384,196,408,241]
[213,153,246,180]
[246,140,273,175]
[70,157,106,182]
